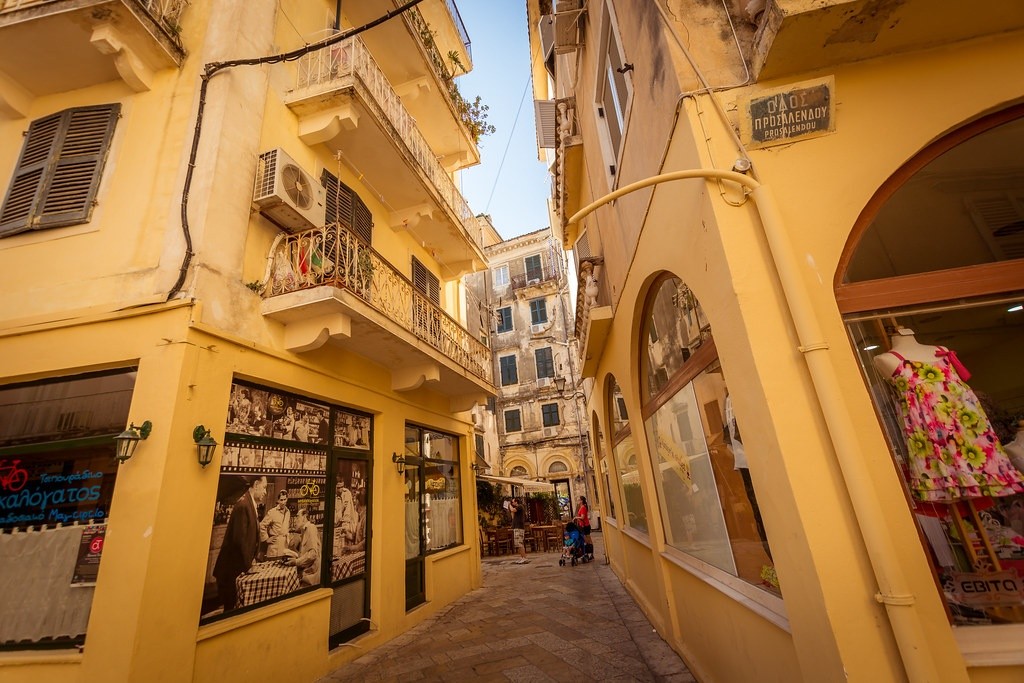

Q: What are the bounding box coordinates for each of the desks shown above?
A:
[332,551,366,581]
[236,558,299,611]
[532,525,557,552]
[485,529,515,556]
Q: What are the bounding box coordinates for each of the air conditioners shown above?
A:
[553,0,578,47]
[531,324,544,334]
[254,148,326,234]
[528,278,540,285]
[542,426,555,437]
[537,377,551,387]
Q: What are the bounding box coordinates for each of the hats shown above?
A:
[564,532,570,536]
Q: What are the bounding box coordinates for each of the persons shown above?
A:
[563,532,576,556]
[508,498,529,564]
[212,384,372,613]
[572,496,595,561]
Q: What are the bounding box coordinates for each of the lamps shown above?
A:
[392,453,405,475]
[554,374,587,403]
[471,463,481,477]
[113,421,151,464]
[192,425,217,468]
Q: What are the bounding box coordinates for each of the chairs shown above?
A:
[477,522,567,557]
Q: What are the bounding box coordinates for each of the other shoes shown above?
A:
[589,555,594,560]
[515,558,529,564]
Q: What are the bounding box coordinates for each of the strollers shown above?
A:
[561,528,590,568]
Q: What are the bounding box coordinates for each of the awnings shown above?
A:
[476,474,555,493]
